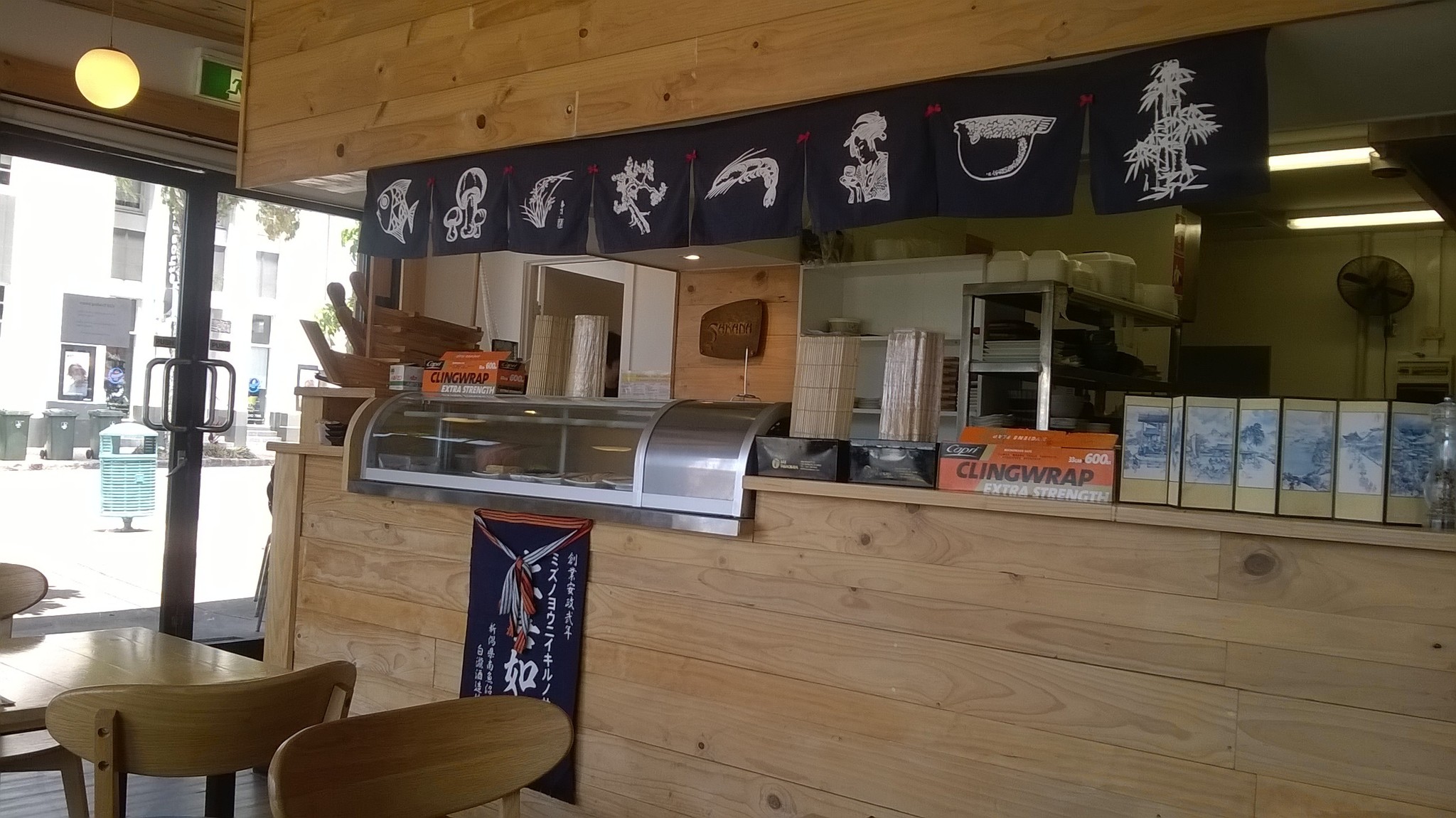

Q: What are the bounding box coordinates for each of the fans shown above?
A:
[1336,254,1417,319]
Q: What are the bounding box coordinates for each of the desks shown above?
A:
[1,627,300,818]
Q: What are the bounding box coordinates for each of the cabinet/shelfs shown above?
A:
[960,281,1179,442]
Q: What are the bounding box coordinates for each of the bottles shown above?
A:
[1423,397,1456,531]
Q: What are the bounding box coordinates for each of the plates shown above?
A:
[472,468,633,491]
[325,423,349,445]
[965,341,1111,435]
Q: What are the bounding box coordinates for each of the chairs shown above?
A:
[266,696,577,818]
[2,560,91,818]
[46,659,359,818]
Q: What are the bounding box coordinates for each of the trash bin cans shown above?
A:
[86,409,123,460]
[98,417,158,533]
[42,407,78,461]
[0,409,34,461]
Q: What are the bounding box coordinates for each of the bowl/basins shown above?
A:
[988,248,1178,314]
